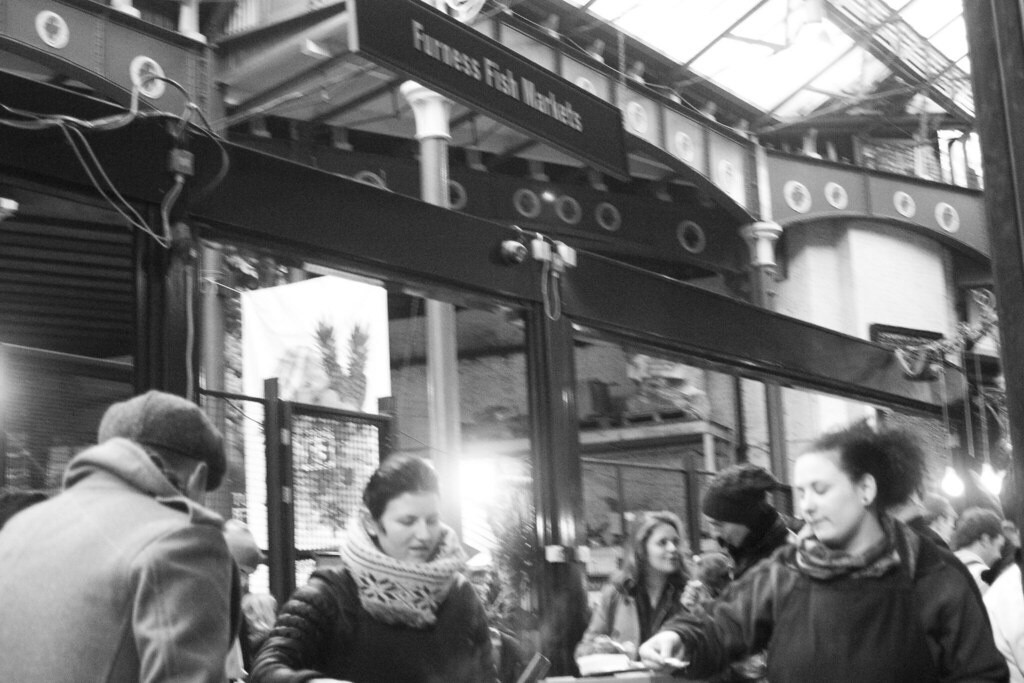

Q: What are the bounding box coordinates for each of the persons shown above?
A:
[250,453,502,683]
[573,421,1024,683]
[0,392,242,683]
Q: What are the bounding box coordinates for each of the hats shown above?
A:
[702,460,790,522]
[96,390,229,496]
